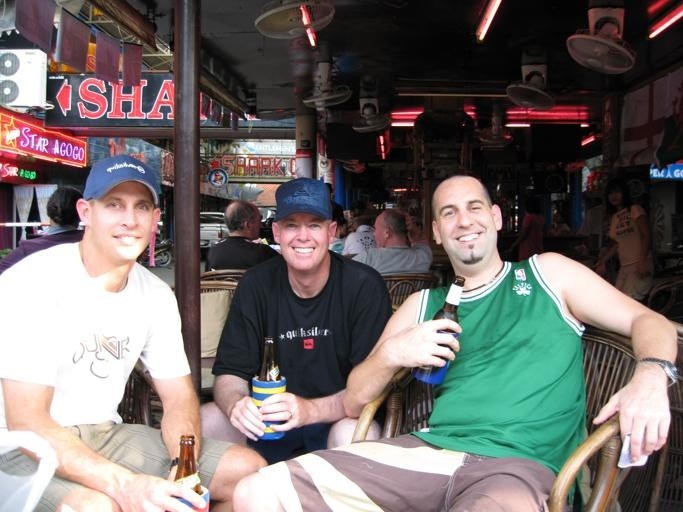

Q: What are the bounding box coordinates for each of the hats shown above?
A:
[272,177,334,222]
[84,154,162,205]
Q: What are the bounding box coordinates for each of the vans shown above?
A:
[200,212,229,247]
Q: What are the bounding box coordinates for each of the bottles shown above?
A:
[170,434,203,499]
[412,275,465,385]
[251,334,286,441]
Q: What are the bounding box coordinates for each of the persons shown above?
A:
[502,194,545,262]
[355,209,433,276]
[206,200,281,282]
[2,153,269,512]
[0,187,84,273]
[341,216,378,256]
[200,179,390,457]
[595,179,654,301]
[234,175,678,512]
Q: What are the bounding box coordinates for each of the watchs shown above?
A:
[638,357,678,388]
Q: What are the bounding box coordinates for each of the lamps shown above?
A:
[475,0,502,43]
[296,5,317,47]
[648,4,683,39]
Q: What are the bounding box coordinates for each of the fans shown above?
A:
[566,9,636,74]
[254,0,336,39]
[507,64,555,110]
[302,56,351,107]
[351,97,391,137]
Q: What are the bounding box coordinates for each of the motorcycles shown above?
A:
[144,239,174,267]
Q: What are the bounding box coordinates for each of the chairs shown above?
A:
[171,281,250,395]
[198,270,248,280]
[645,278,683,323]
[383,272,440,312]
[353,327,671,512]
